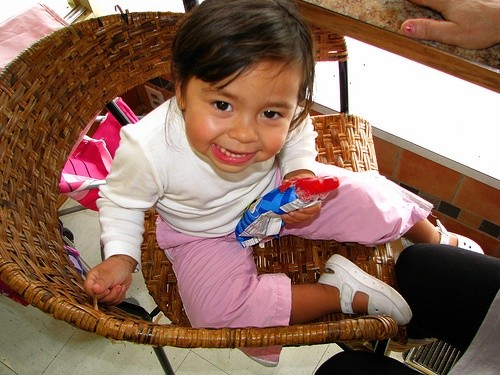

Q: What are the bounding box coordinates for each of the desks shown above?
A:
[288,0,500,94]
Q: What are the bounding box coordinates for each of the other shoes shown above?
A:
[318,254,413,326]
[402,219,485,256]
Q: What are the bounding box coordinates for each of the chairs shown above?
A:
[0,11,409,375]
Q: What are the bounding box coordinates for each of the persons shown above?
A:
[315,0,500,375]
[84,0,484,367]
